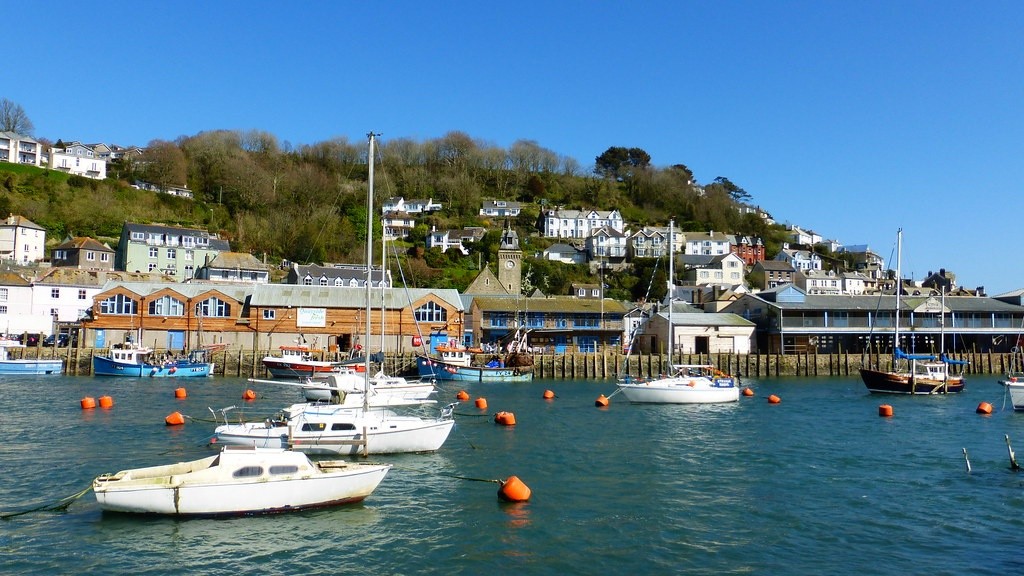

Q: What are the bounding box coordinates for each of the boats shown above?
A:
[93,443,392,519]
[998,317,1024,412]
[0,338,64,377]
[413,323,539,382]
[94,324,214,380]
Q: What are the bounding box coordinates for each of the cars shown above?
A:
[17,334,48,346]
[43,333,71,347]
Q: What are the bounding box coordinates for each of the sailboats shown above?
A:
[207,131,473,457]
[613,219,749,403]
[852,227,972,396]
